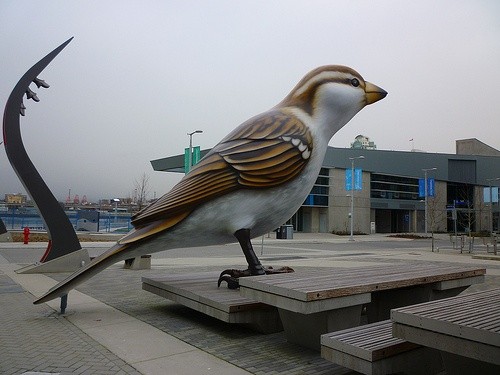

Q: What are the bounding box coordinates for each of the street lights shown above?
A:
[486,177,500,235]
[186,129,203,172]
[348,155,365,242]
[422,167,437,238]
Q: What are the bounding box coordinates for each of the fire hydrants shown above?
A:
[23,226,30,245]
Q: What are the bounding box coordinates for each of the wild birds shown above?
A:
[33,64,388,315]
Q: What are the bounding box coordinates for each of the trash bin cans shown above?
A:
[286,224,294,239]
[276,225,287,239]
